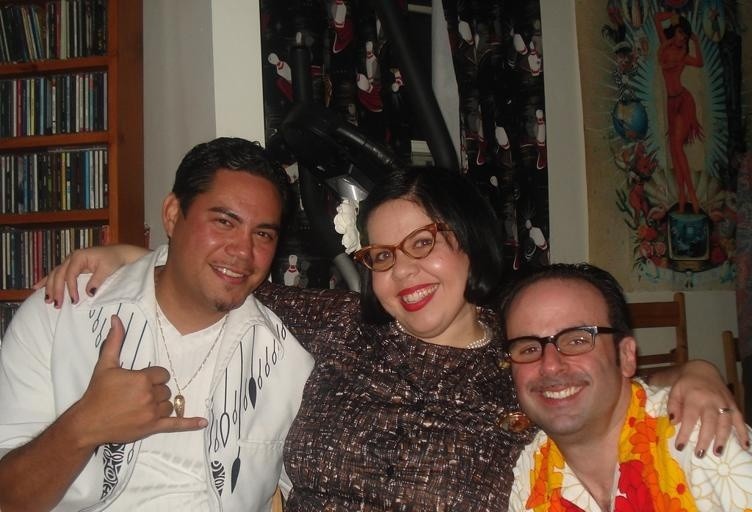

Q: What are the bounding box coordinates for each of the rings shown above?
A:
[718,407,730,416]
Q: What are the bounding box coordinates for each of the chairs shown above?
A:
[629,288,692,412]
[722,331,750,412]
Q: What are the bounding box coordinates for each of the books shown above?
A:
[0,0,110,340]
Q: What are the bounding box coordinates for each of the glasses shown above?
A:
[506,325,617,364]
[354,223,461,273]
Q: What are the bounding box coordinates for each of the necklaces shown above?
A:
[394,320,496,348]
[154,311,229,420]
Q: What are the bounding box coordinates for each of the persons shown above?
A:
[501,262,749,512]
[654,11,704,214]
[0,136,317,512]
[30,165,752,512]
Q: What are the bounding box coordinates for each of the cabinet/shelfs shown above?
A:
[1,1,146,350]
